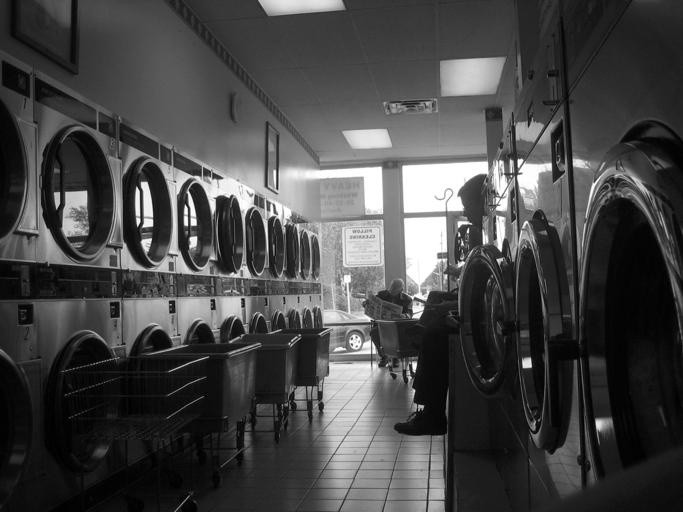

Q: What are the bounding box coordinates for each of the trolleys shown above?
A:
[372,315,420,384]
[55,356,199,509]
[228,333,295,442]
[143,342,264,486]
[267,326,332,415]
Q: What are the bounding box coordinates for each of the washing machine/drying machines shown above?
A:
[0,47,324,512]
[442,0,683,512]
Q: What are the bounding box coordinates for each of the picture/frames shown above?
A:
[11,0,80,75]
[264,121,279,196]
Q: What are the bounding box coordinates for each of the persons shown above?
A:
[361,278,413,369]
[389,173,491,436]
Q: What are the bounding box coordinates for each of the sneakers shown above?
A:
[378,357,391,367]
[394,412,447,435]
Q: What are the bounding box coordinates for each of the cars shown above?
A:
[316,307,372,353]
[350,291,365,298]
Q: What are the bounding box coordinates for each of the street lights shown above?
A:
[342,273,352,313]
